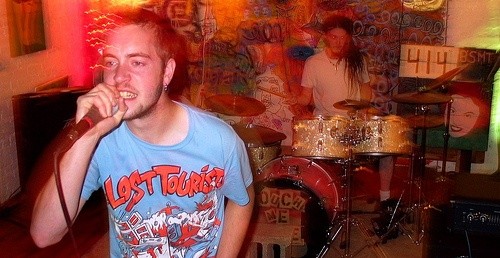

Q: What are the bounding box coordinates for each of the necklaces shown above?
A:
[325,49,345,70]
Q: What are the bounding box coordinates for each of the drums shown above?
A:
[252,157,341,229]
[248,126,282,174]
[293,115,351,162]
[353,114,413,157]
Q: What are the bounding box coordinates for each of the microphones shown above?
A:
[55,104,118,155]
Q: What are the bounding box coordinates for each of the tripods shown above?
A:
[318,103,444,258]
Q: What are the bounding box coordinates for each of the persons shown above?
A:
[32,9,256,258]
[280,14,410,225]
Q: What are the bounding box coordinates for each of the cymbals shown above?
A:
[390,91,452,106]
[228,124,288,147]
[404,113,443,129]
[333,99,379,111]
[207,93,266,117]
[421,64,472,94]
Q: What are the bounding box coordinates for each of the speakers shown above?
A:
[444,200,500,258]
